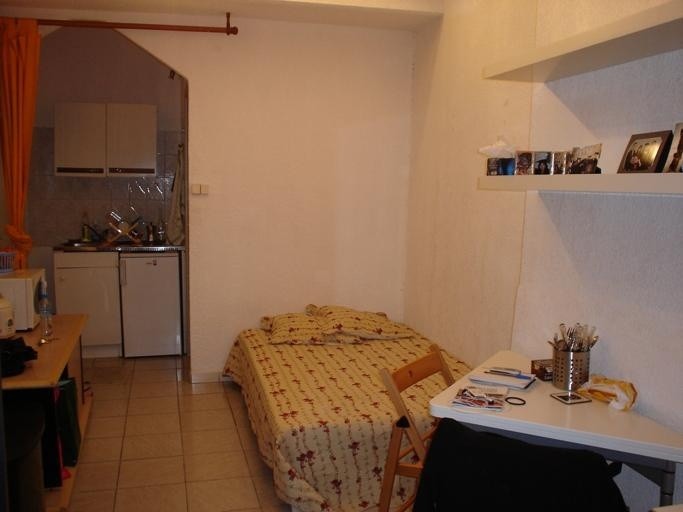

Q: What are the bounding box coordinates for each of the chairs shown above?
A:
[379,343,456,511]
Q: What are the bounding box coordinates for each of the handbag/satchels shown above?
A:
[48,377,81,467]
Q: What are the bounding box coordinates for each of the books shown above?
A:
[467,368,539,390]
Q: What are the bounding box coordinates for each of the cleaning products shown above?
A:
[81,210,90,242]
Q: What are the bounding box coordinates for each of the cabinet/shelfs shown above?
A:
[54,253,122,360]
[477,0,683,194]
[119,253,183,358]
[53,101,107,178]
[106,103,157,177]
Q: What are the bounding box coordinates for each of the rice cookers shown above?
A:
[0,298,16,338]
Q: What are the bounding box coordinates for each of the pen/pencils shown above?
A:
[484,370,531,379]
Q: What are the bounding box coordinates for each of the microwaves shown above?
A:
[0,268,47,331]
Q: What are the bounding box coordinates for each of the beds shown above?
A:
[222,304,473,512]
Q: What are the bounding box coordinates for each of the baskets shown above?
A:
[553,350,590,391]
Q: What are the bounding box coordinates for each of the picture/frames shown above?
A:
[663,123,683,173]
[616,130,671,173]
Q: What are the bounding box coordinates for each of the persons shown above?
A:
[534,160,549,175]
[626,139,657,171]
[567,152,599,173]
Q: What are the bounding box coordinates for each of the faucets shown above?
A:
[80,222,100,239]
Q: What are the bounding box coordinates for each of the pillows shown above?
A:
[305,304,416,340]
[261,313,364,345]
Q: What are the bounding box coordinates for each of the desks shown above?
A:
[413,350,682,512]
[1,313,94,511]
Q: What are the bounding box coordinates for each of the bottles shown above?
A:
[83,225,89,240]
[38,294,54,337]
[157,221,166,245]
[148,222,154,242]
